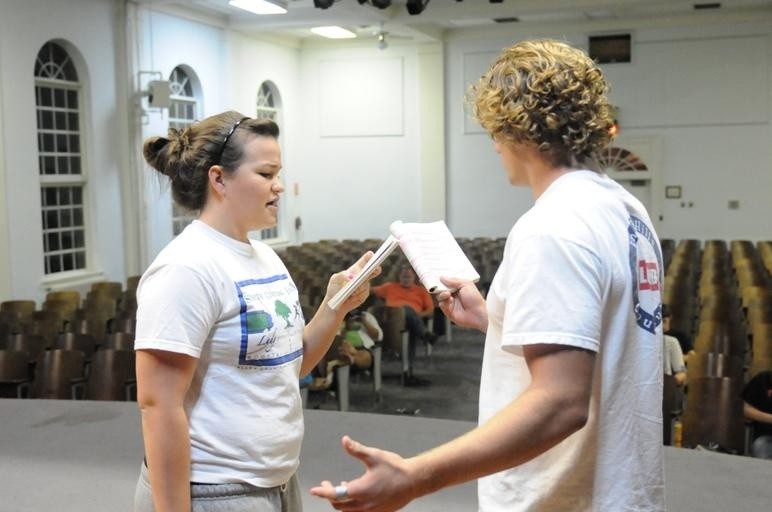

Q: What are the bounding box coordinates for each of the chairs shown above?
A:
[0,237,509,413]
[656,235,771,454]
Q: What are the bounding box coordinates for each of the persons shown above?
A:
[371,264,436,384]
[662,335,685,444]
[741,370,772,461]
[307,41,665,512]
[132,111,384,512]
[328,302,383,399]
[664,305,693,385]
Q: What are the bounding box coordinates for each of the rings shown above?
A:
[334,486,349,504]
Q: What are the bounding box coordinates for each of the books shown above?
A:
[326,234,401,311]
[391,220,481,295]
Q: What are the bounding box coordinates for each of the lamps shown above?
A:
[373,29,389,49]
